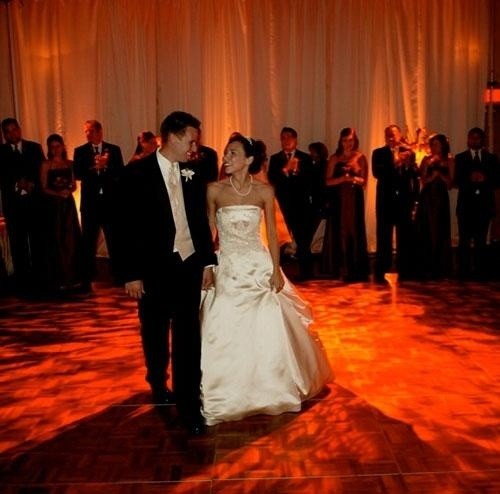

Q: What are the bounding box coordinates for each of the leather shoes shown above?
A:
[151,386,172,405]
[185,412,204,433]
[375,273,388,284]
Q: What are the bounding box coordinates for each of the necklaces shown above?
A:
[228,174,255,196]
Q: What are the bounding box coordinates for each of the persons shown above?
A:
[268,127,319,282]
[452,126,498,283]
[325,127,373,281]
[195,128,219,184]
[72,121,126,290]
[128,130,162,164]
[418,133,455,282]
[197,130,337,428]
[105,109,219,438]
[306,139,343,280]
[40,133,87,284]
[0,115,50,300]
[371,124,421,285]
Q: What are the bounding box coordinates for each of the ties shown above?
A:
[474,151,479,161]
[288,153,293,175]
[14,145,21,194]
[169,164,195,263]
[95,147,102,192]
